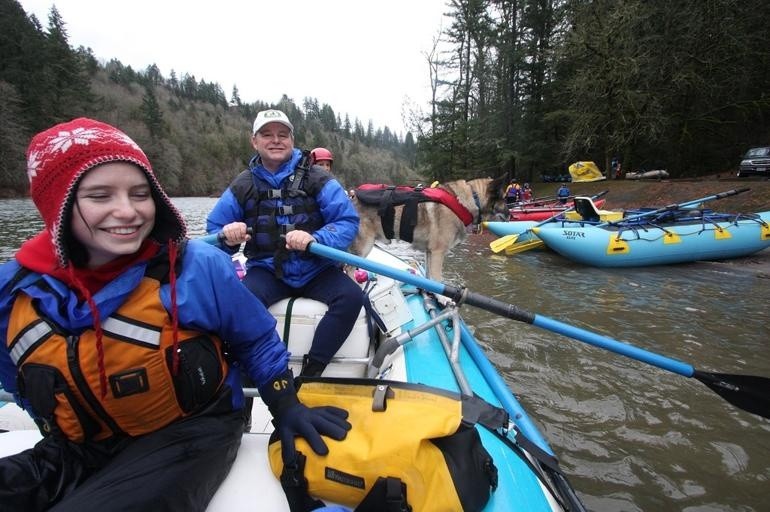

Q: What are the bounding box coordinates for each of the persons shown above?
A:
[556,182,571,205]
[0,117,353,512]
[309,145,334,174]
[615,158,623,180]
[501,177,522,219]
[522,182,534,201]
[204,109,363,380]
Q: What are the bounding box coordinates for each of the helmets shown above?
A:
[311,148,333,167]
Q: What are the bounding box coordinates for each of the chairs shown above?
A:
[573,197,615,226]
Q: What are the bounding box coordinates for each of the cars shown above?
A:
[738,147,770,176]
[541,174,572,183]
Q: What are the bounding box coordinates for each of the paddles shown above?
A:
[490,189,612,252]
[277,230,770,418]
[506,186,752,255]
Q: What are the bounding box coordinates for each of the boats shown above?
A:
[624,168,670,180]
[479,195,770,269]
[2,236,584,512]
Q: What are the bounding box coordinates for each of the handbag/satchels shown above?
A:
[251,378,499,512]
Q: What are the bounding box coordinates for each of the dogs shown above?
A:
[342,170,514,283]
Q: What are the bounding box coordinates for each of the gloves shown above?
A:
[260,371,352,464]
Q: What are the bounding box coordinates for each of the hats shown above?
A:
[252,108,295,137]
[26,117,187,268]
